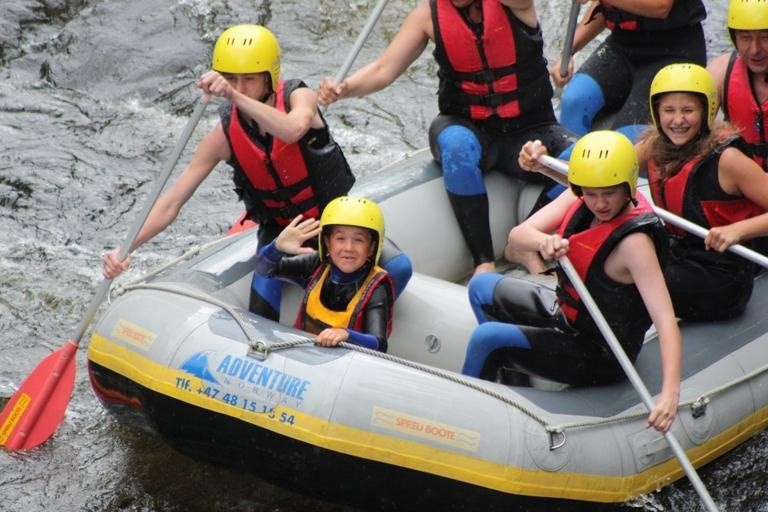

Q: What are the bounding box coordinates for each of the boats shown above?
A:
[86,107,768,511]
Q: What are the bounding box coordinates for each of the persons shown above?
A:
[526,1,709,221]
[256,195,394,355]
[516,62,768,324]
[318,2,583,274]
[103,24,413,324]
[462,128,681,433]
[696,0,768,171]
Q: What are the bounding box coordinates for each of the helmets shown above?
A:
[649,64,718,141]
[318,196,384,266]
[727,1,767,53]
[212,24,280,92]
[567,130,640,200]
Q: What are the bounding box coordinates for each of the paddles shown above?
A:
[1,92,213,450]
[227,0,388,235]
